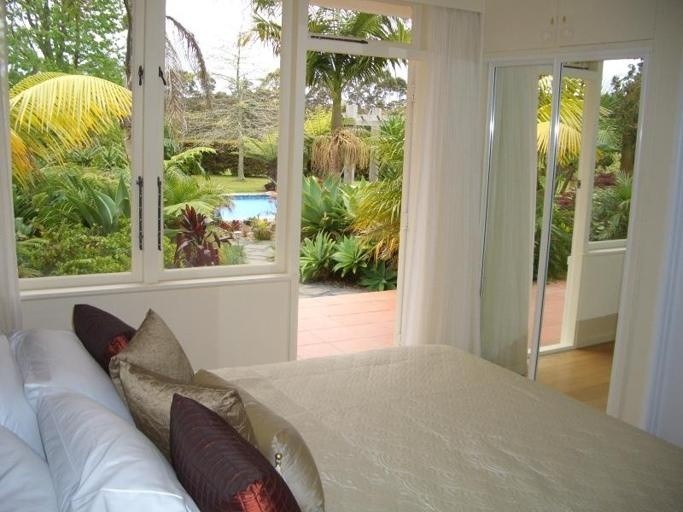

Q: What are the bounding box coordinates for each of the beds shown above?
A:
[0,339,683,511]
